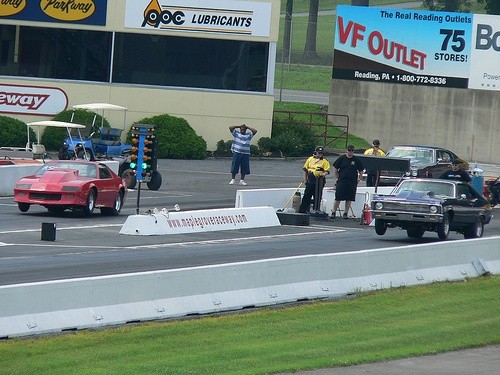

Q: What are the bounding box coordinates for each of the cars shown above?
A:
[13,159,127,217]
[370,178,494,241]
[361,144,470,187]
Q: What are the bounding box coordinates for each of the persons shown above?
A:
[363,139,386,187]
[228,124,257,186]
[439,160,473,185]
[330,144,364,219]
[302,146,331,211]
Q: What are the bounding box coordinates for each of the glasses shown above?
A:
[452,165,457,166]
[348,150,354,152]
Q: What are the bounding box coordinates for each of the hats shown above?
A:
[315,146,324,152]
[373,140,380,146]
[452,160,459,164]
[348,145,355,149]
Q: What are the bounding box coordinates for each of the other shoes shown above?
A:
[330,212,336,218]
[343,213,347,218]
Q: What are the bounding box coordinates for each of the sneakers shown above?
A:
[239,180,247,185]
[229,179,236,185]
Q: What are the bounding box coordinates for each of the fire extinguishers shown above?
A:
[363,203,371,224]
